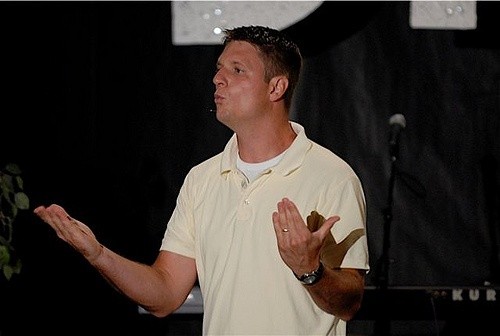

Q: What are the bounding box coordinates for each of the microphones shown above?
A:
[387,113,407,158]
[209,109,216,112]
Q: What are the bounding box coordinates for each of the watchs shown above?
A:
[293,261,325,285]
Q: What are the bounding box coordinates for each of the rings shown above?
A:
[281,228,289,232]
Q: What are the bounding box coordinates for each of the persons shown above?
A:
[33,24,371,336]
